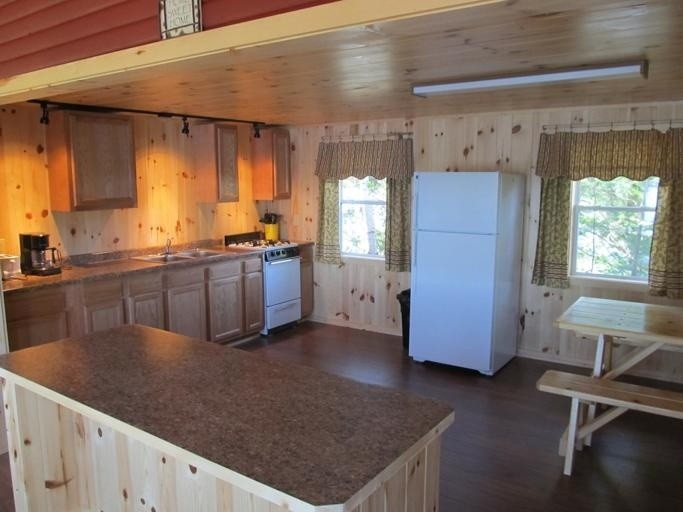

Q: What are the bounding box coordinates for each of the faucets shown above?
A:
[158,239,176,256]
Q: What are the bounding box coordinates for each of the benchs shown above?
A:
[575,331,682,410]
[536,369,683,478]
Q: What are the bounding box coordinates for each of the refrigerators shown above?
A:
[407,170,525,376]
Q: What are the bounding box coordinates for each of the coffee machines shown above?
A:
[20,233,63,275]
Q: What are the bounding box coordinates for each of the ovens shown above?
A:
[259,255,302,336]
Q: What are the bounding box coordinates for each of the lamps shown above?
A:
[253,121,261,139]
[408,58,647,99]
[39,102,50,122]
[181,116,193,135]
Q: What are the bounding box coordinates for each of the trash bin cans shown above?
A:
[396,288,411,348]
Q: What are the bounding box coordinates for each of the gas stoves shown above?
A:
[228,239,298,251]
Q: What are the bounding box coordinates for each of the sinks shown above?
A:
[134,253,189,266]
[180,248,218,262]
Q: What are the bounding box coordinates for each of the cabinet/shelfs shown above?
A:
[301,245,314,321]
[207,257,243,346]
[194,123,241,204]
[77,278,124,334]
[45,107,139,212]
[243,256,265,337]
[4,284,76,352]
[124,265,166,331]
[250,125,292,203]
[161,266,208,342]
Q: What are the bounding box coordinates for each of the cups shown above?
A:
[0,254,18,280]
[264,222,279,242]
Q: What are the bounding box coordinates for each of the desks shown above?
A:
[553,297,683,448]
